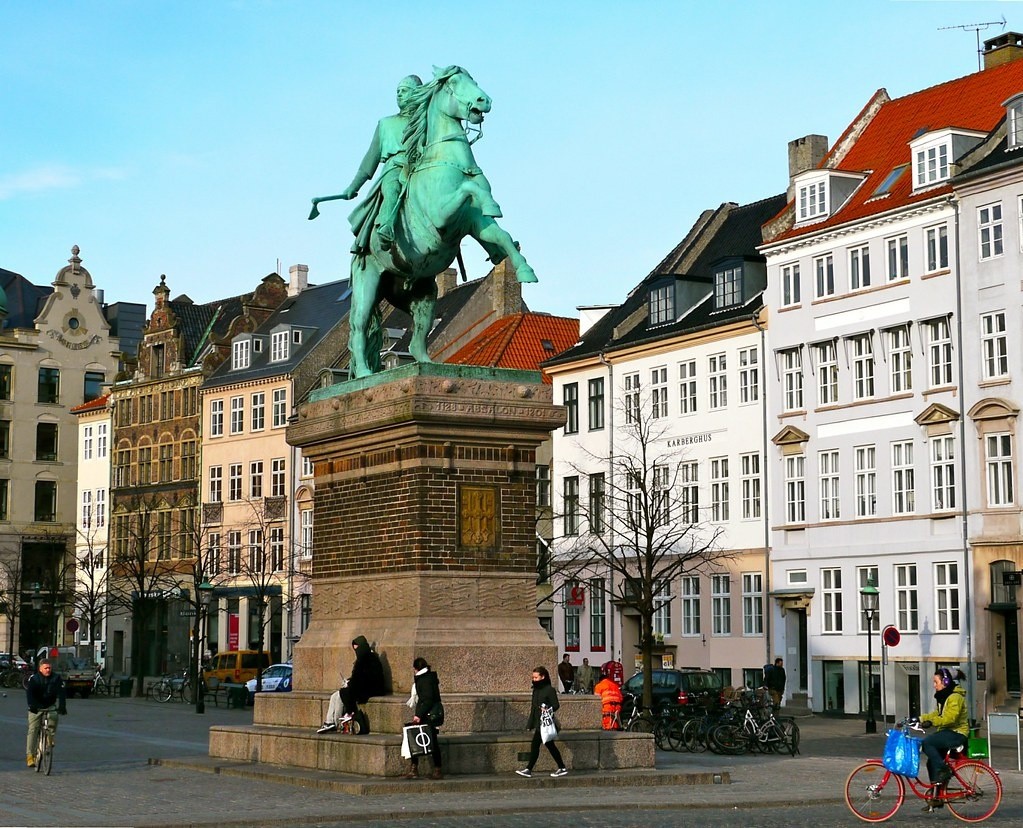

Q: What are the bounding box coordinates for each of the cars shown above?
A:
[0,653,27,669]
[243,662,293,706]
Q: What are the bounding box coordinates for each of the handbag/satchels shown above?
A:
[882,725,923,778]
[540,711,557,745]
[400,722,434,759]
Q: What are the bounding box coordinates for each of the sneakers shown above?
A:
[550,768,568,777]
[516,769,532,778]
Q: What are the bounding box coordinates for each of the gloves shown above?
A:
[58,707,67,715]
[908,717,920,724]
[29,705,38,715]
[922,721,932,728]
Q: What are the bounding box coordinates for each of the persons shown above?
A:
[558,654,575,693]
[343,75,519,265]
[592,674,623,731]
[908,667,970,812]
[764,658,786,715]
[398,658,446,779]
[316,635,385,733]
[577,658,595,694]
[515,666,568,777]
[27,659,68,767]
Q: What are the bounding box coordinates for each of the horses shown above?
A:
[348,63,538,375]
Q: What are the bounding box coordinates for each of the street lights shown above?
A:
[198,576,214,714]
[30,583,45,661]
[859,573,877,732]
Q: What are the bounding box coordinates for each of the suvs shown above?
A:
[621,668,725,723]
[201,650,272,693]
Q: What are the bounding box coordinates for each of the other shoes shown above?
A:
[47,736,54,747]
[936,766,950,781]
[922,800,944,812]
[339,712,354,724]
[26,754,35,767]
[316,722,335,733]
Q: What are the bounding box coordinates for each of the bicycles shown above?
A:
[151,666,192,703]
[602,686,801,754]
[28,709,58,776]
[844,718,1003,823]
[0,666,35,690]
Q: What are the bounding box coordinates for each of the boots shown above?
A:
[401,764,418,779]
[430,768,442,779]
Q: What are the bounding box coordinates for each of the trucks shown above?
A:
[31,647,94,699]
